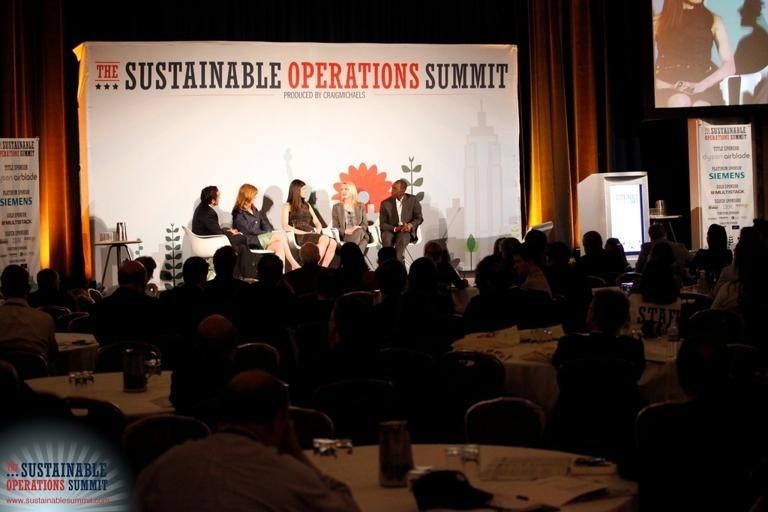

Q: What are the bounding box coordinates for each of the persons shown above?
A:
[1,181,768,512]
[653,0,736,108]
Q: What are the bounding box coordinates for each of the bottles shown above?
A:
[122,345,158,392]
[377,419,414,488]
[668,321,680,360]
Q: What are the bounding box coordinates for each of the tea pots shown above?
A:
[117,222,127,242]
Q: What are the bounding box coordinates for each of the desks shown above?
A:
[651,211,683,240]
[1,287,682,512]
[93,239,143,285]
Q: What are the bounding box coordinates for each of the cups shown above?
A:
[68,369,97,418]
[405,463,435,507]
[311,437,353,479]
[443,444,481,480]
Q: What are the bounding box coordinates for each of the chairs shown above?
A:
[184,227,231,274]
[247,231,283,253]
[678,293,714,331]
[286,229,334,250]
[332,228,379,271]
[375,215,422,262]
